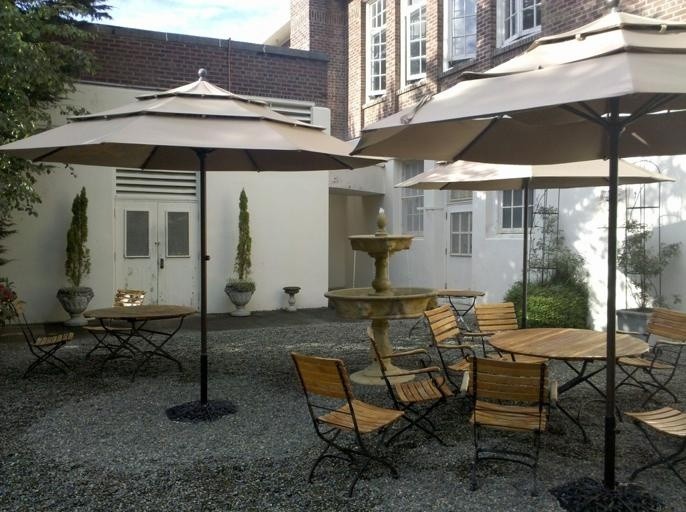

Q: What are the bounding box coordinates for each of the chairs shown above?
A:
[286,348,398,497]
[459,355,559,497]
[616,306,686,407]
[422,302,499,401]
[12,298,75,379]
[84,288,146,360]
[472,301,551,363]
[364,324,456,448]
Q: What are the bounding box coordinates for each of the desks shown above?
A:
[82,304,199,385]
[485,326,651,445]
[436,290,486,332]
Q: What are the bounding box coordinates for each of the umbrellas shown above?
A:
[350,7,686,490]
[0,68,396,407]
[393,160,677,329]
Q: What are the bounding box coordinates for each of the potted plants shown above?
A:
[223,186,257,316]
[54,186,95,328]
[616,219,683,348]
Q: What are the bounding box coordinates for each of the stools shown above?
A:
[620,405,686,486]
